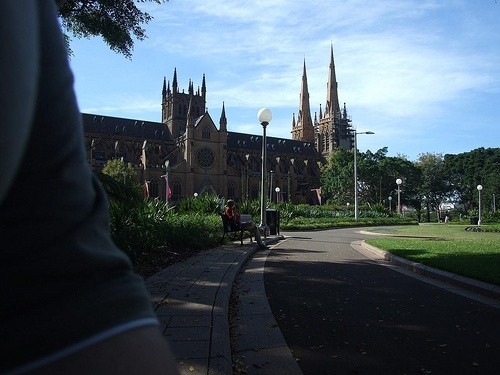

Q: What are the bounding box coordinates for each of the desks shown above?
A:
[396,179,403,213]
[476,185,483,225]
[353,130,375,221]
[194,193,198,203]
[258,108,272,239]
[388,196,393,213]
[275,187,281,205]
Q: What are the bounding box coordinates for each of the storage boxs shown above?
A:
[218,211,268,246]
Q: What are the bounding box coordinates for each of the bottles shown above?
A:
[227,199,235,207]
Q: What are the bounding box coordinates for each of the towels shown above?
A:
[266,209,279,235]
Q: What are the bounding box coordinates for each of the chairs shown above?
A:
[258,245,271,250]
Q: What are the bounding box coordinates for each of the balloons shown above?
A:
[195,147,217,169]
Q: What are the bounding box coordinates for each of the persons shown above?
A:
[225,199,270,250]
[1,0,181,374]
